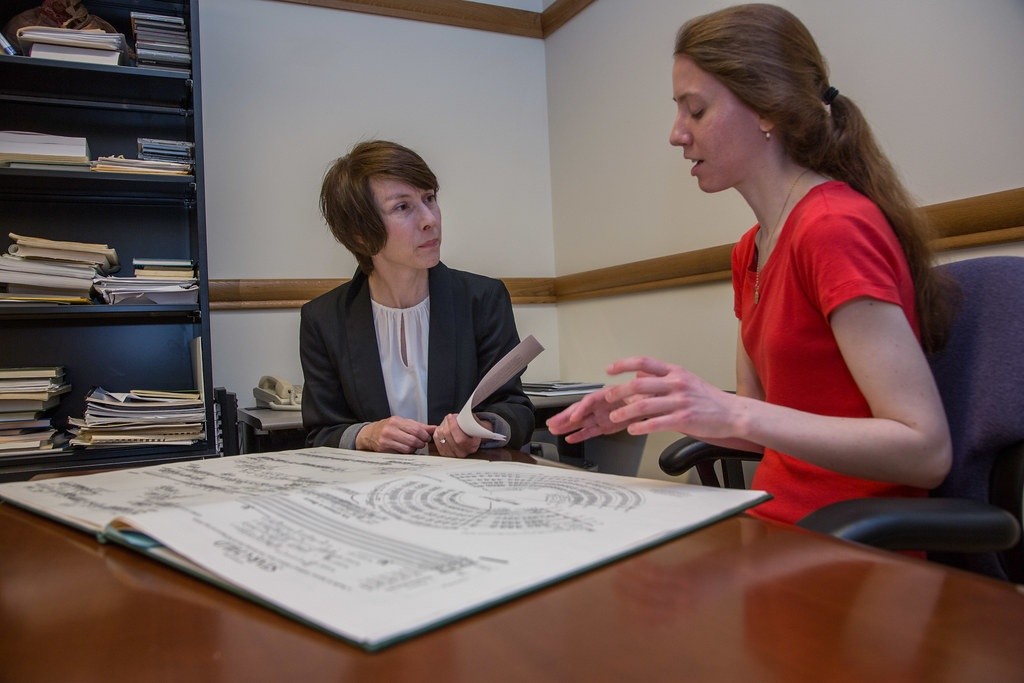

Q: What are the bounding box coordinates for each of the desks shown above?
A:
[0,449,1023,683]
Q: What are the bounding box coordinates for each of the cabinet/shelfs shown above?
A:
[0,1,217,483]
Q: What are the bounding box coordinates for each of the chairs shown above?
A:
[659,254,1024,588]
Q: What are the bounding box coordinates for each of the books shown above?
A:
[0,337,206,457]
[0,12,192,74]
[0,447,774,651]
[522,381,604,397]
[0,233,198,306]
[0,130,195,176]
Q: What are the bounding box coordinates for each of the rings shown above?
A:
[440,439,446,444]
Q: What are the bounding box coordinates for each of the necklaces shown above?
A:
[754,169,809,304]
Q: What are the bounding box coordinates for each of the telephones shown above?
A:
[253,375,304,412]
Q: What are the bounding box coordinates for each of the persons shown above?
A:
[546,2,952,562]
[300,140,535,459]
